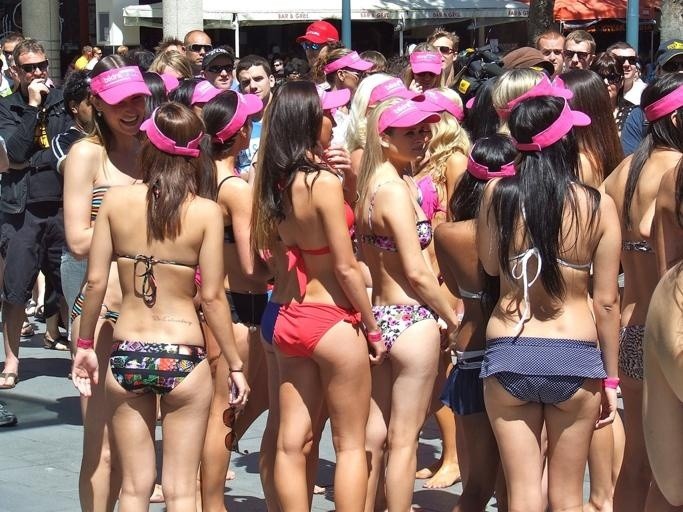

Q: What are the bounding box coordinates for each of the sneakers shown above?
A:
[0,404,17,426]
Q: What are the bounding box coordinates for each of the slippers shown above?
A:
[20,323,35,337]
[25,303,37,316]
[0,371,20,389]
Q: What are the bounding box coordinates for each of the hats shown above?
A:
[654,39,683,67]
[315,83,351,110]
[323,50,374,75]
[139,106,203,158]
[146,71,179,96]
[644,83,683,120]
[511,95,591,151]
[410,51,442,75]
[202,48,233,70]
[495,71,573,121]
[378,98,440,135]
[501,46,555,75]
[89,66,152,105]
[190,79,223,106]
[466,133,518,179]
[296,20,339,44]
[416,89,464,120]
[369,77,425,105]
[210,88,263,143]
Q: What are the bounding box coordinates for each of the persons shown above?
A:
[534,31,563,78]
[200,48,233,89]
[154,39,187,57]
[0,60,18,427]
[499,47,556,82]
[360,51,382,77]
[0,38,73,390]
[73,102,250,512]
[253,77,388,512]
[585,52,645,147]
[434,143,511,512]
[234,55,274,183]
[78,46,93,72]
[465,76,499,143]
[427,27,462,90]
[117,44,125,59]
[183,31,213,78]
[597,72,683,512]
[292,22,339,102]
[355,98,465,511]
[199,91,270,511]
[271,56,285,87]
[562,30,596,70]
[415,86,468,490]
[220,46,239,90]
[309,49,374,151]
[123,46,155,72]
[65,53,164,504]
[164,78,225,120]
[405,43,437,94]
[477,95,612,512]
[493,69,573,135]
[85,47,102,70]
[284,62,302,80]
[50,68,94,345]
[560,70,626,512]
[608,41,647,108]
[155,51,192,89]
[643,156,683,508]
[635,56,644,81]
[620,38,683,158]
[262,224,327,512]
[0,33,54,97]
[344,73,425,285]
[141,71,179,125]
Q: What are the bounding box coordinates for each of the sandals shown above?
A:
[43,332,69,351]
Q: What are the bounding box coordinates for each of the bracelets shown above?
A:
[351,192,359,209]
[76,337,94,349]
[365,328,382,342]
[602,378,621,389]
[229,369,243,372]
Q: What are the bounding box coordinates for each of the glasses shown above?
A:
[207,64,233,73]
[274,65,279,66]
[223,404,248,458]
[290,72,299,75]
[96,53,101,54]
[186,44,212,53]
[599,73,623,83]
[562,49,591,61]
[436,46,456,54]
[19,59,48,72]
[662,61,683,72]
[302,42,320,50]
[617,56,636,65]
[2,50,13,55]
